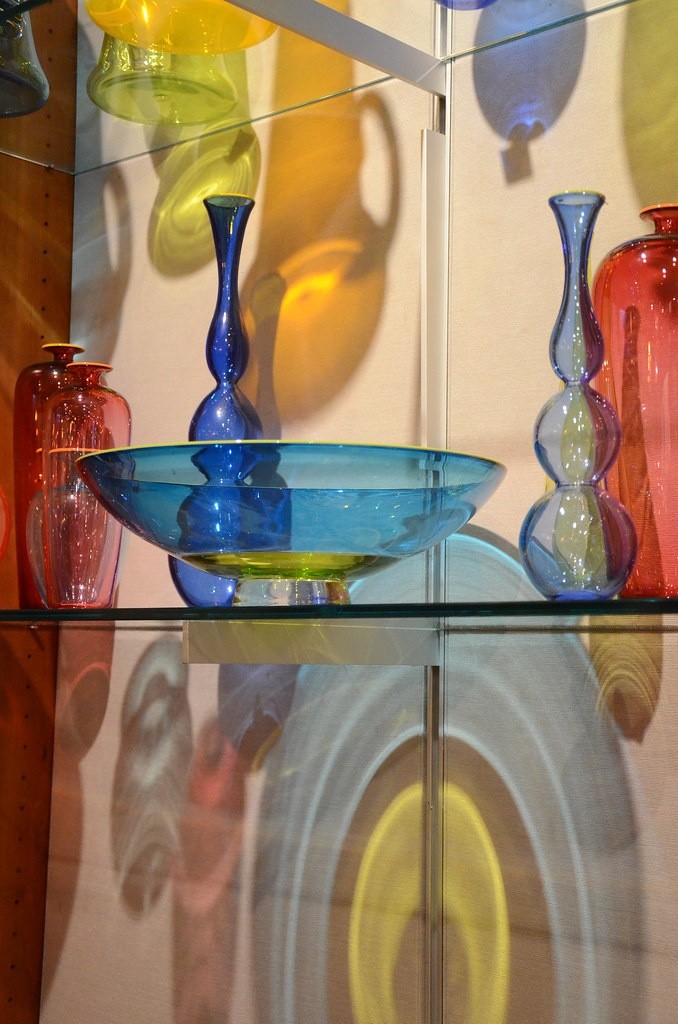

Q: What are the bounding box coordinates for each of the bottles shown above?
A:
[0,0,677,610]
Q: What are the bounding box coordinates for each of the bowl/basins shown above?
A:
[73,440,506,606]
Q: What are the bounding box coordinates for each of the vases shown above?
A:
[590,202,677,598]
[13,343,132,608]
